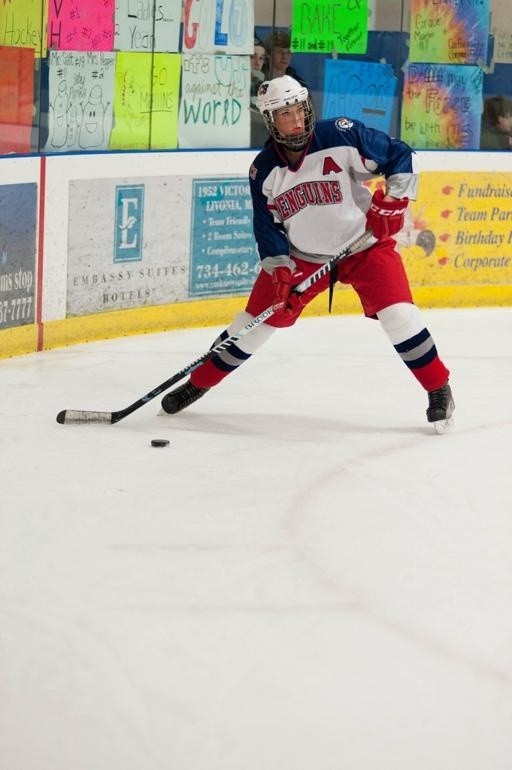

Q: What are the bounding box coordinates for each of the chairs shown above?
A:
[162,379,209,414]
[426,378,455,422]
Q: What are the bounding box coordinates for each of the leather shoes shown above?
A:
[365,189,409,242]
[270,266,303,319]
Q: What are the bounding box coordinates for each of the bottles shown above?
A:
[254,31,265,55]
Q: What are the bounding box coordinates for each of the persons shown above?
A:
[251,33,264,98]
[162,75,456,422]
[479,95,512,150]
[262,32,309,85]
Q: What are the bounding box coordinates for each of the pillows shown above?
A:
[256,74,315,147]
